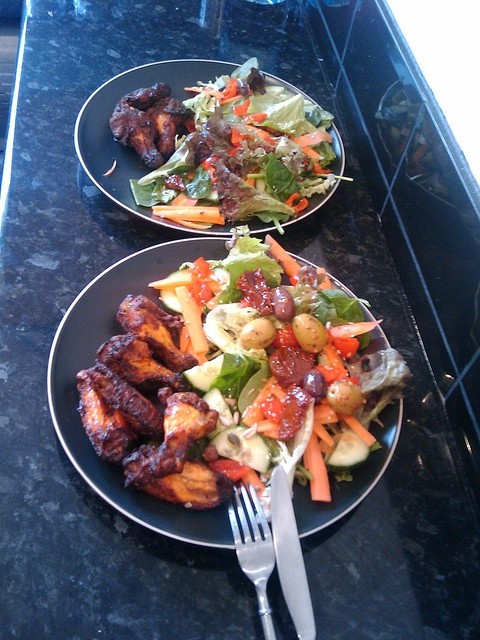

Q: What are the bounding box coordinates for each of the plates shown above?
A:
[73,58,346,234]
[47,238,404,548]
[377,77,479,215]
[76,153,175,231]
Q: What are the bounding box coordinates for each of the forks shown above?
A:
[228,481,276,639]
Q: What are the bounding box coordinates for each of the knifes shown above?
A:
[270,461,317,639]
